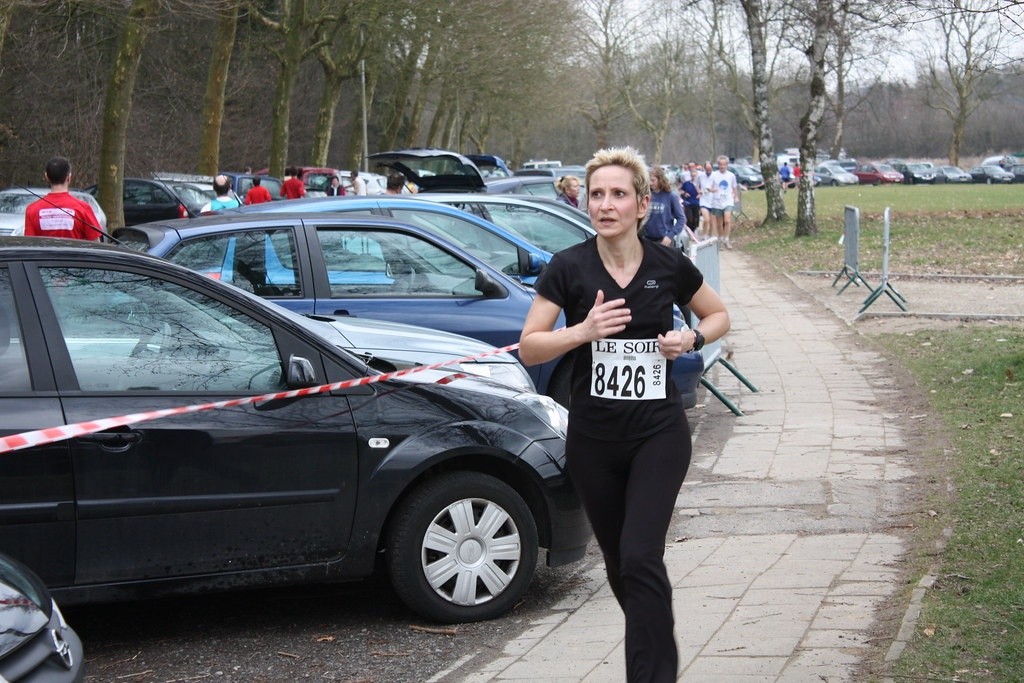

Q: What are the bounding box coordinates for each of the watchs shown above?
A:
[686,329,705,354]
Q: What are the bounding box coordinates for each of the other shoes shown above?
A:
[718,238,724,251]
[725,240,731,250]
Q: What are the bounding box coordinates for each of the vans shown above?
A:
[777,156,811,166]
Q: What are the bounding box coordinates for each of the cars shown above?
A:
[788,169,821,187]
[814,165,859,187]
[853,163,904,185]
[108,212,705,413]
[0,235,596,621]
[931,166,974,184]
[821,160,857,171]
[893,163,937,184]
[0,287,536,395]
[0,553,87,682]
[0,188,107,240]
[981,156,1024,182]
[86,173,287,224]
[969,166,1015,184]
[259,148,764,253]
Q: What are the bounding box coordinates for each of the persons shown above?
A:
[488,166,505,177]
[244,175,272,205]
[401,170,419,194]
[676,155,739,251]
[793,164,801,188]
[199,174,241,214]
[518,143,731,683]
[779,162,790,182]
[382,171,406,195]
[350,169,366,196]
[556,176,580,208]
[327,176,345,196]
[639,166,686,247]
[25,156,103,241]
[280,166,306,200]
